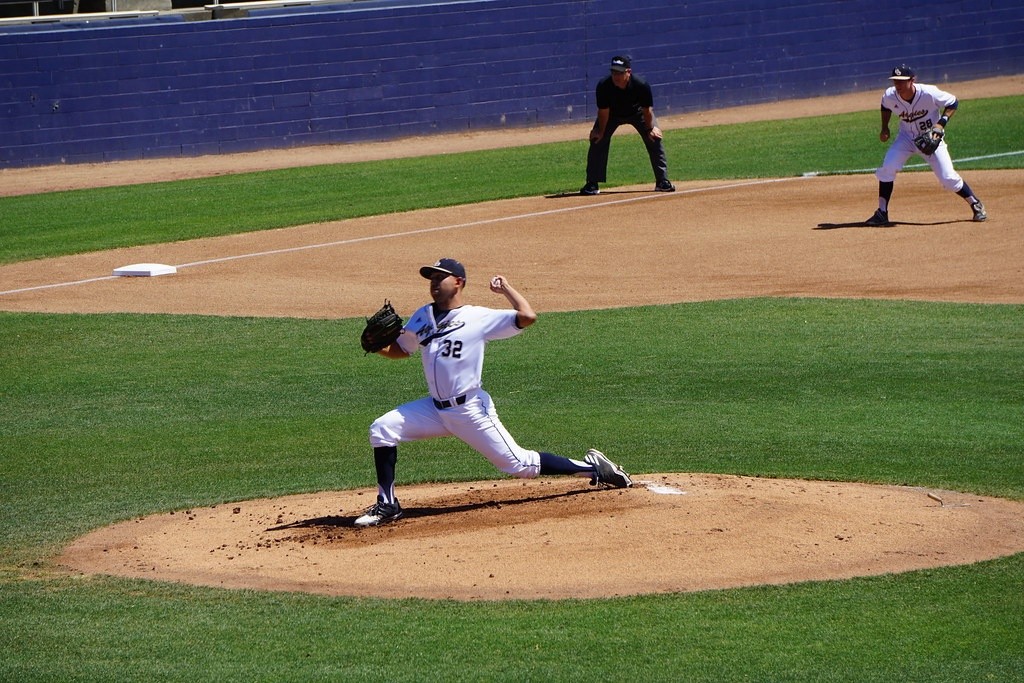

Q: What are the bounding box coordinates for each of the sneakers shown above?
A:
[580,182,600,194]
[655,178,675,191]
[865,208,889,225]
[971,201,986,221]
[583,448,633,488]
[352,495,403,526]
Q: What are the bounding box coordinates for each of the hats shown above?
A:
[888,64,915,80]
[608,55,631,72]
[420,258,466,281]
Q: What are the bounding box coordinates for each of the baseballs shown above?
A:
[491,276,500,285]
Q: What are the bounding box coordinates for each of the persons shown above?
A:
[866,63,987,225]
[353,258,633,527]
[580,56,675,194]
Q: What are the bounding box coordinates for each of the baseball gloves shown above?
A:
[911,125,945,159]
[360,298,407,357]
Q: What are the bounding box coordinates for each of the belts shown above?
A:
[432,395,466,410]
